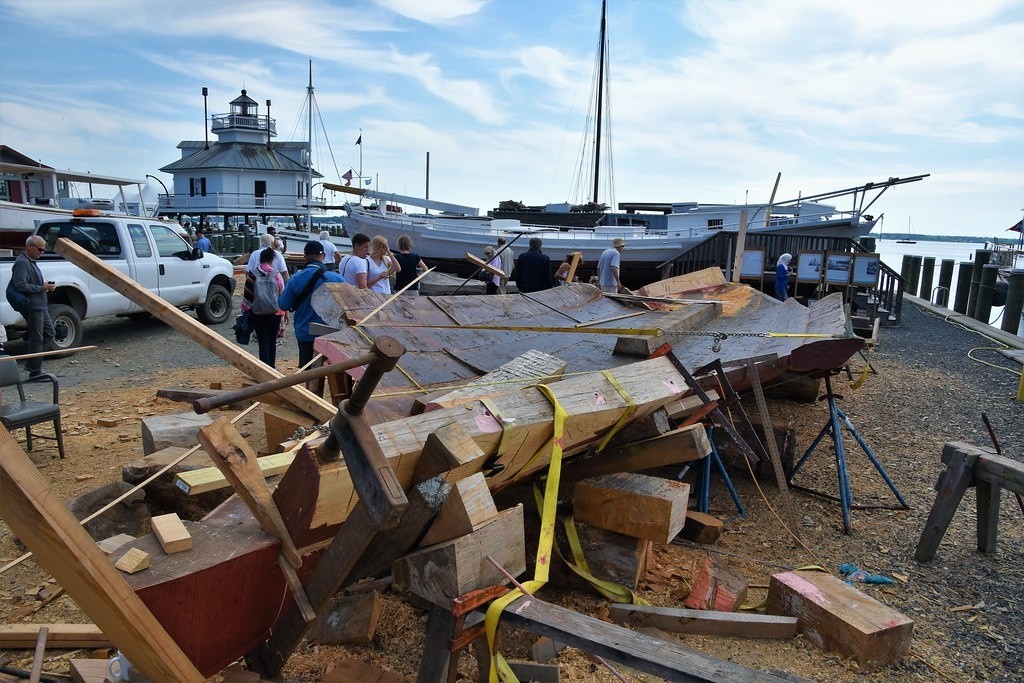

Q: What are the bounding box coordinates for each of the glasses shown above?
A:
[621,246,625,248]
[485,252,491,255]
[269,232,276,235]
[31,243,44,253]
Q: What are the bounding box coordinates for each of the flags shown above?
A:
[1006,219,1024,234]
[355,134,362,145]
[342,170,352,186]
[365,179,371,185]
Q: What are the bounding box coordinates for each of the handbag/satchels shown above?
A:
[208,243,216,254]
[233,316,255,345]
[6,281,30,311]
[478,270,493,282]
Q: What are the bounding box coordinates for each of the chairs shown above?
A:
[0,355,65,459]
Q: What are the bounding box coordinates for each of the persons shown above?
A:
[388,235,429,296]
[184,222,213,252]
[496,237,515,294]
[339,233,371,289]
[555,251,583,285]
[515,238,554,294]
[597,238,627,293]
[363,236,401,294]
[774,253,793,301]
[481,246,502,294]
[267,227,287,254]
[246,234,290,346]
[242,248,290,371]
[11,235,56,382]
[278,241,346,390]
[318,231,342,272]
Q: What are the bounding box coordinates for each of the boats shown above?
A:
[339,200,886,284]
[984,237,1024,320]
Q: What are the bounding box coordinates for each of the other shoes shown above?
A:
[23,364,45,374]
[28,372,55,382]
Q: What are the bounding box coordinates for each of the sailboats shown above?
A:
[256,59,354,256]
[896,216,918,244]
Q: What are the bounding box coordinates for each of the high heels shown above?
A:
[279,329,285,337]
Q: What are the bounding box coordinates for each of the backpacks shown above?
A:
[248,267,279,315]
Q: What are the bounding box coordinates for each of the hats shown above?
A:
[611,238,627,247]
[194,229,203,234]
[304,240,324,254]
[352,234,370,247]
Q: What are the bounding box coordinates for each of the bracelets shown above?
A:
[389,253,394,256]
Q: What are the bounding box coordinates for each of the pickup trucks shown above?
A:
[0,208,236,357]
